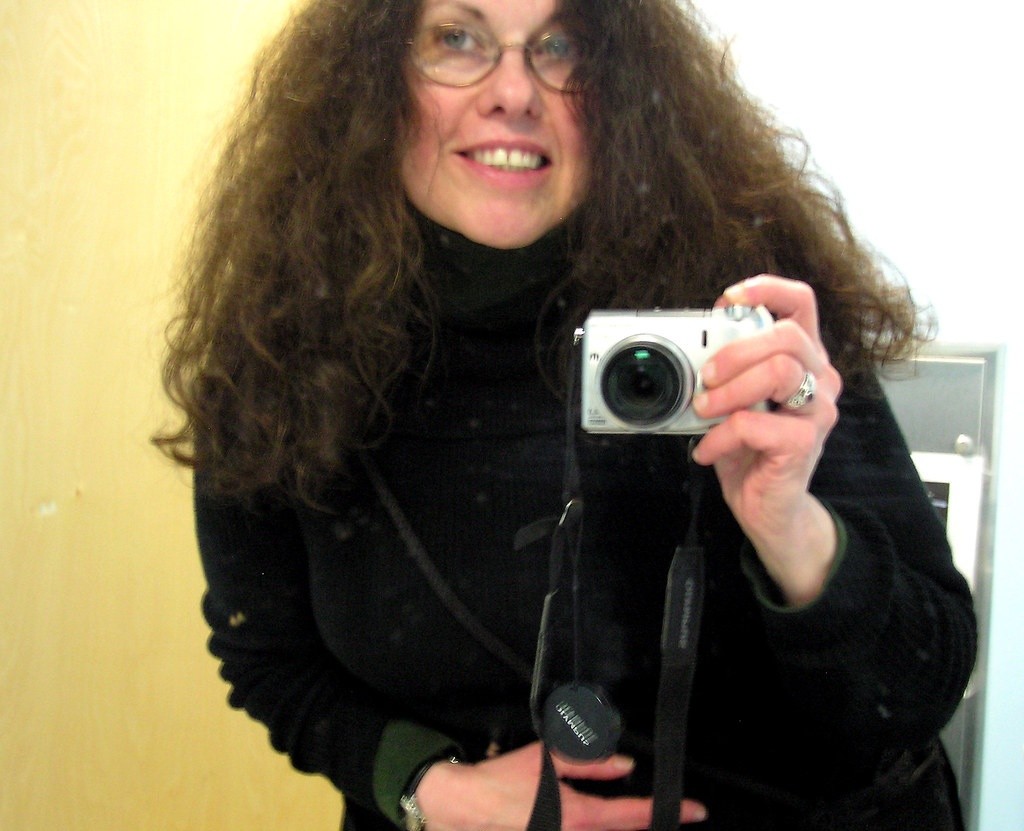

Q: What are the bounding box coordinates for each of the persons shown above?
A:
[149,0,980,831]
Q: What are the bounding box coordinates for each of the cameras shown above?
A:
[575,301,774,433]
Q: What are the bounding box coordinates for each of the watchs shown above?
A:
[400,755,459,831]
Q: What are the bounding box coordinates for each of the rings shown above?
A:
[782,372,816,411]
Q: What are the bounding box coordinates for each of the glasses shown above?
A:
[400,21,590,93]
